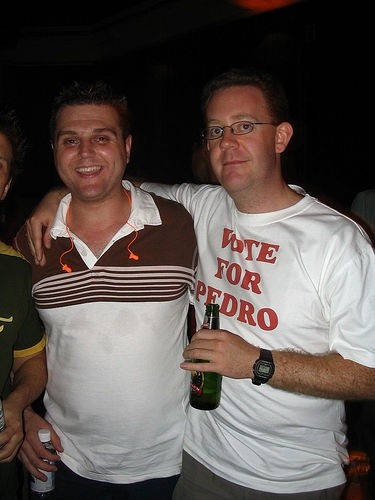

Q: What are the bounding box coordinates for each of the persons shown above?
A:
[192,127,375,500]
[0,132,49,500]
[11,85,198,500]
[25,74,375,500]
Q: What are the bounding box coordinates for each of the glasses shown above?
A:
[200,119,274,140]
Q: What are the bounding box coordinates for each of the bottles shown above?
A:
[189,303,222,411]
[0,399,7,450]
[29,428,55,500]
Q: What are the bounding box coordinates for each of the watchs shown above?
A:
[252,349,276,386]
